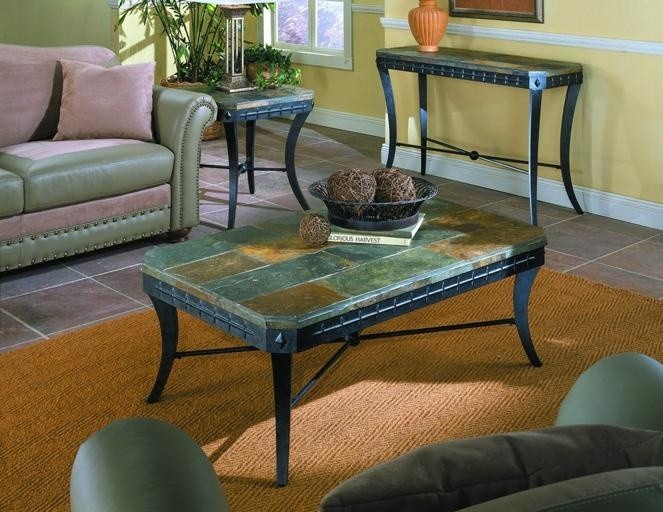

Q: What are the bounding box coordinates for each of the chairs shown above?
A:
[70,351,663,512]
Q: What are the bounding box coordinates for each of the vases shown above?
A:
[408,0,448,53]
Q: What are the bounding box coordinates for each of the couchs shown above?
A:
[0,43,218,273]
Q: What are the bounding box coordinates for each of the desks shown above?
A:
[199,83,315,230]
[376,46,583,227]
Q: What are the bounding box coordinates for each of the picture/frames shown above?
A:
[450,0,544,23]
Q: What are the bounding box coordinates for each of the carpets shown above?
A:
[0,267,663,512]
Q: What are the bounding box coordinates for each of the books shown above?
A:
[325,211,426,247]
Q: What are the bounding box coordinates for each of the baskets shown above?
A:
[309,166,438,231]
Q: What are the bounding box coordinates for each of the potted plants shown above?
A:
[109,0,301,93]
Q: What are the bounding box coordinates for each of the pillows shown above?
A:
[320,424,663,512]
[54,58,156,141]
